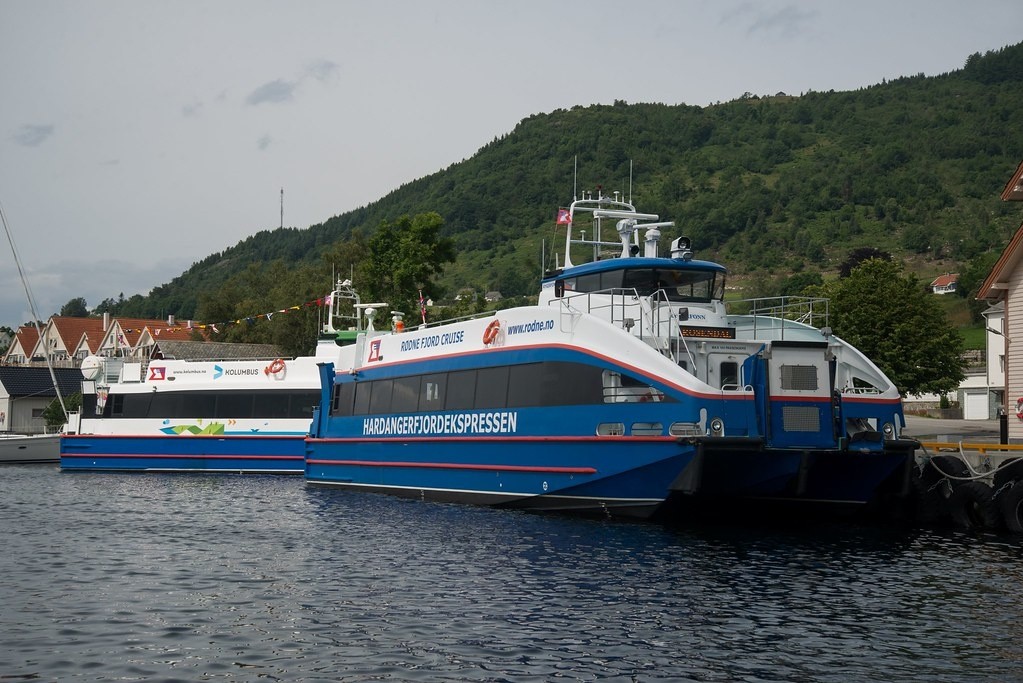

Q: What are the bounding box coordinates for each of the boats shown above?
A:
[60,357,339,474]
[305,191,921,526]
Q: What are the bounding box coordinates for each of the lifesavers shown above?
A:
[482,319,500,345]
[1015,397,1023,419]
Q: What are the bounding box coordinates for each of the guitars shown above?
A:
[269,359,285,374]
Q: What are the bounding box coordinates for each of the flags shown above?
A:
[208,313,274,334]
[420,293,427,317]
[557,210,572,224]
[117,334,127,348]
[280,299,322,313]
[126,325,205,336]
